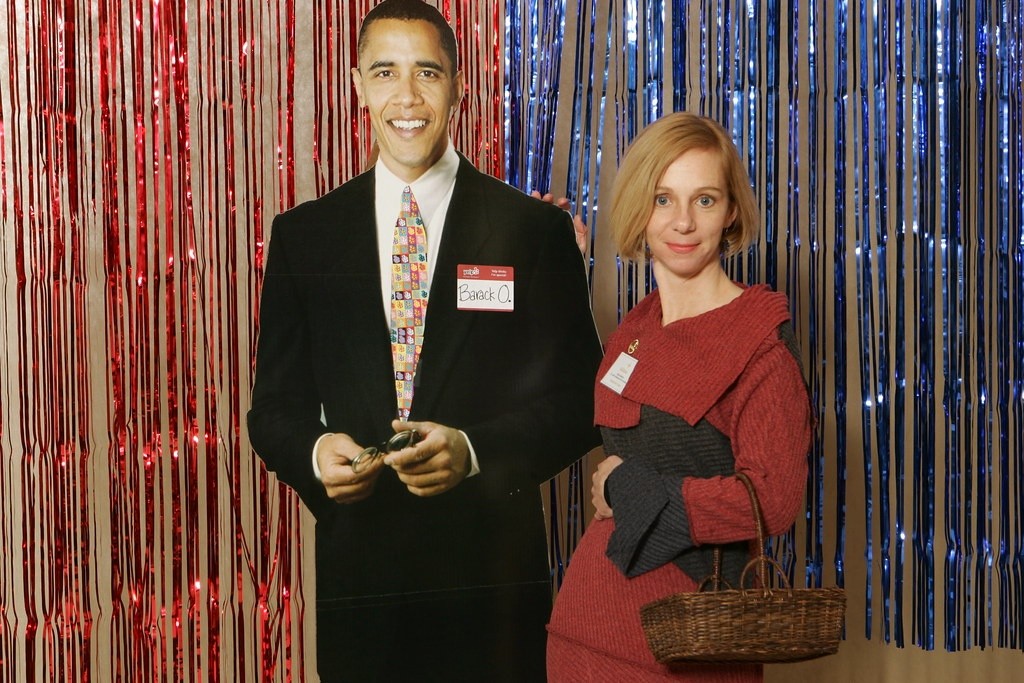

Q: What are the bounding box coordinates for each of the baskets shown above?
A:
[641,472,847,664]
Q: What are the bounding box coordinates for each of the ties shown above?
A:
[390,186,429,422]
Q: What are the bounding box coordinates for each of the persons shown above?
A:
[247,1,601,683]
[529,111,812,683]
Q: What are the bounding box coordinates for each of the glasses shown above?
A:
[352,429,422,473]
